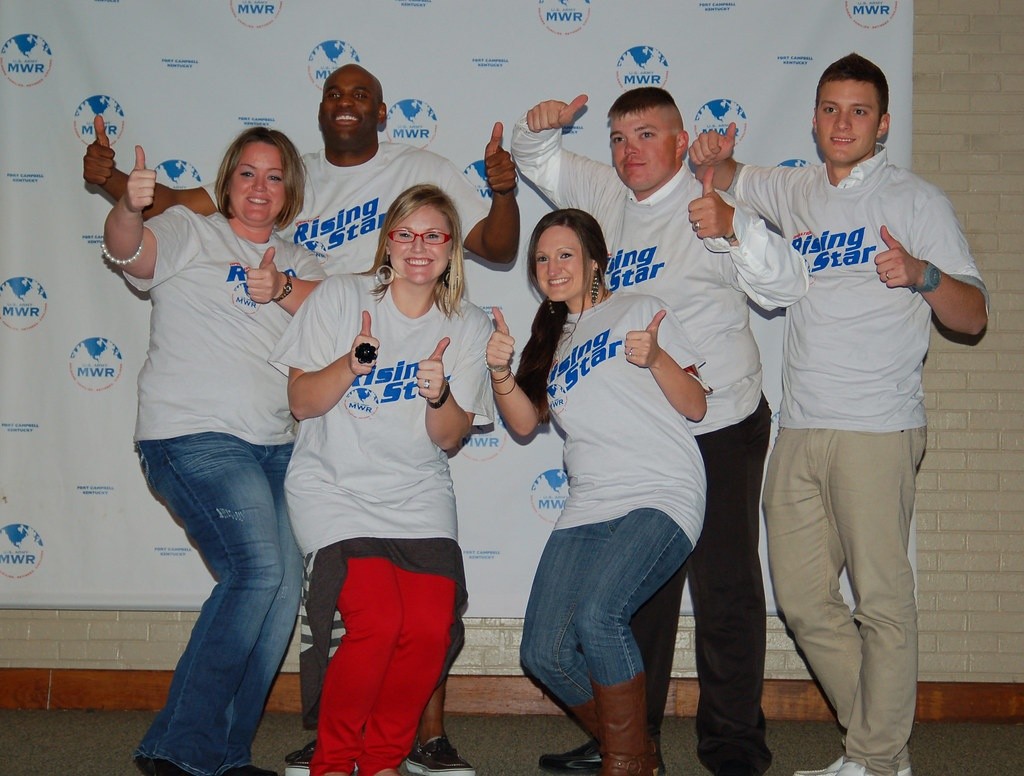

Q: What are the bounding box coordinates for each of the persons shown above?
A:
[687,50,990,776]
[509,86,809,776]
[84,64,521,776]
[486,208,707,776]
[102,125,331,776]
[266,185,495,776]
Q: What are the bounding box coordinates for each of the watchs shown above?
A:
[910,259,941,293]
[273,272,292,302]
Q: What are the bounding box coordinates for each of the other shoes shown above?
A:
[217,764,280,776]
[133,754,194,776]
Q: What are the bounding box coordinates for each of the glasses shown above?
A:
[387,230,452,245]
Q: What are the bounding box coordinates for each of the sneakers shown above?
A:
[285,738,358,776]
[406,735,477,776]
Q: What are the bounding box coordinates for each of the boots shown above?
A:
[569,671,659,776]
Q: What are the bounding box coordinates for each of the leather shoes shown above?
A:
[793,756,912,776]
[538,739,603,773]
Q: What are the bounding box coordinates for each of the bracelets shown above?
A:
[425,379,450,409]
[484,354,515,395]
[100,241,143,265]
[724,208,737,242]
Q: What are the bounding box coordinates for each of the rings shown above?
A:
[354,343,377,364]
[425,380,429,388]
[693,222,700,230]
[886,272,890,279]
[630,349,632,354]
